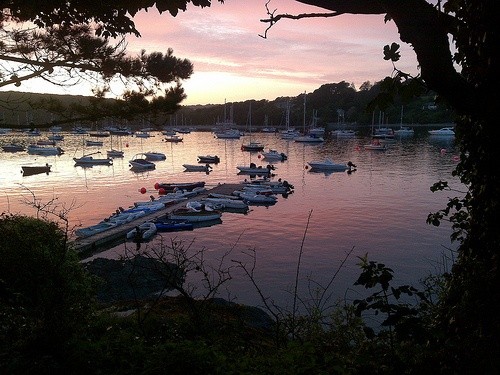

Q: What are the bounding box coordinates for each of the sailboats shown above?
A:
[106,124,125,158]
[11,90,457,146]
[240,102,264,152]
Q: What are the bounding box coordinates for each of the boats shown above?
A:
[75,176,295,243]
[196,155,221,165]
[136,151,167,161]
[27,145,65,155]
[129,158,156,173]
[72,151,113,166]
[235,162,276,173]
[261,149,288,162]
[364,138,391,151]
[304,158,357,176]
[182,163,213,170]
[20,163,52,177]
[237,171,279,179]
[1,143,26,153]
[183,169,213,175]
